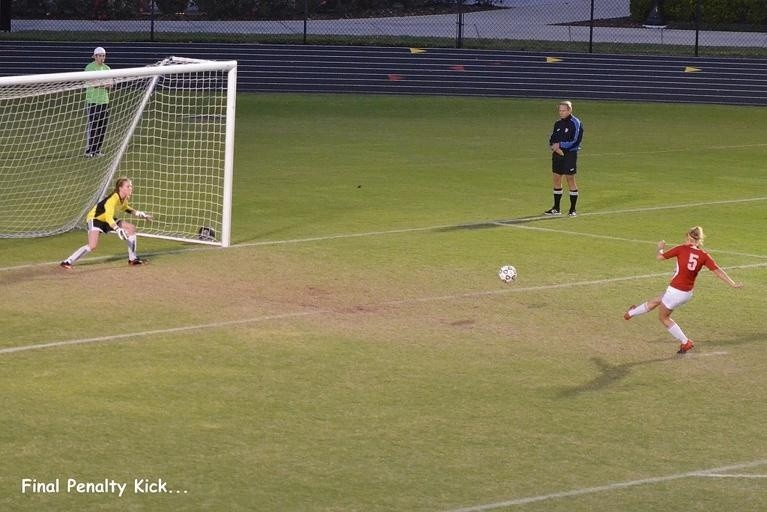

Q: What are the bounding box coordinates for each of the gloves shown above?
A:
[114,226,129,240]
[135,210,153,222]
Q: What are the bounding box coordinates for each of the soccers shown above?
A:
[499,265,517,283]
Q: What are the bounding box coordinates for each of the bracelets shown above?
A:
[655,247,664,256]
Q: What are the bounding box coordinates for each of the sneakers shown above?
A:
[624,305,637,320]
[128,256,149,267]
[60,262,72,269]
[568,209,576,217]
[545,206,562,215]
[677,339,694,354]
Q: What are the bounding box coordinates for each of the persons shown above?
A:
[82,46,113,158]
[57,177,154,270]
[623,226,744,354]
[544,100,583,218]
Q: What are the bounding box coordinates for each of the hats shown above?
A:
[91,47,105,57]
